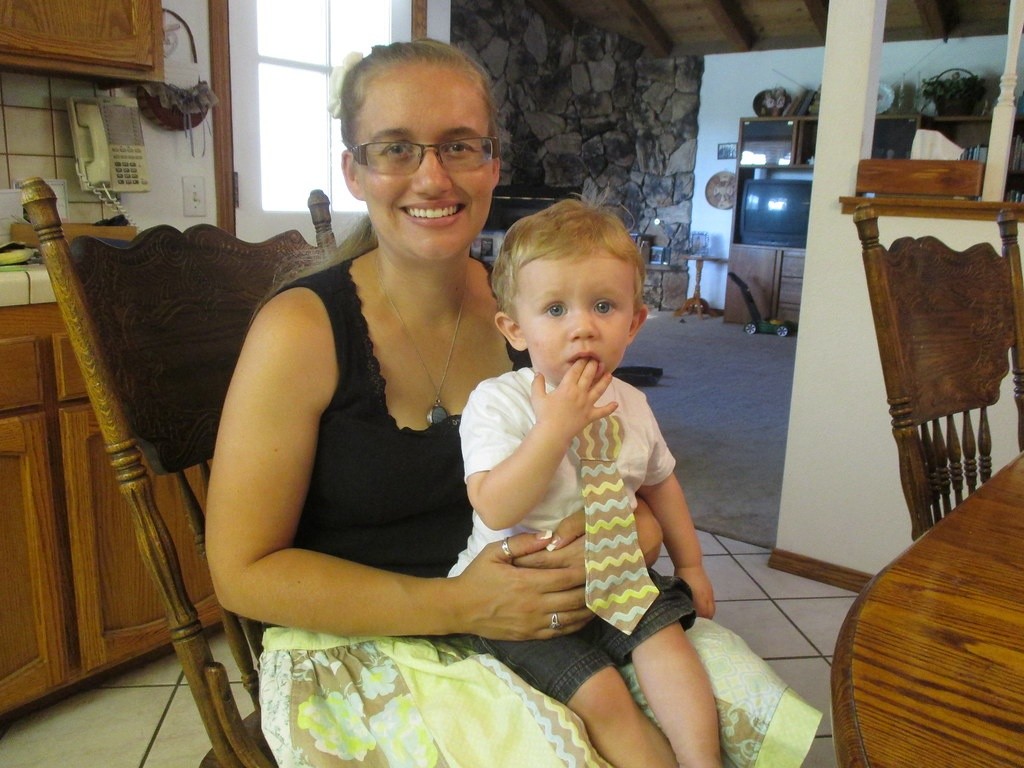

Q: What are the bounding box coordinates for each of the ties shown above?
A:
[578,418,662,635]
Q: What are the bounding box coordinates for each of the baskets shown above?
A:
[925,68,986,116]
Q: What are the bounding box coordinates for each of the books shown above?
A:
[958,134,1024,204]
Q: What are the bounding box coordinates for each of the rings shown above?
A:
[550,613,562,630]
[501,537,513,558]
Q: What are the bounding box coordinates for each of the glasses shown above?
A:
[348,135,503,175]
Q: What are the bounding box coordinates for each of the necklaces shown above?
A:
[376,261,466,428]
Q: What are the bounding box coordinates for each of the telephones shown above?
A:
[66,94,150,193]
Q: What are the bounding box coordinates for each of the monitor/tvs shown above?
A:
[740,179,812,249]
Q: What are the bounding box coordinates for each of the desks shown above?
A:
[674,256,723,320]
[831,451,1024,768]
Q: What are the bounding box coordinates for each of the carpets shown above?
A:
[611,315,797,549]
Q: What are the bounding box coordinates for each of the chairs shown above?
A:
[851,202,1024,540]
[22,177,336,768]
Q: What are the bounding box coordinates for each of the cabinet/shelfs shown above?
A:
[723,113,1024,326]
[0,0,164,90]
[0,301,222,717]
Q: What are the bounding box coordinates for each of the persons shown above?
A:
[204,37,822,768]
[445,198,724,768]
